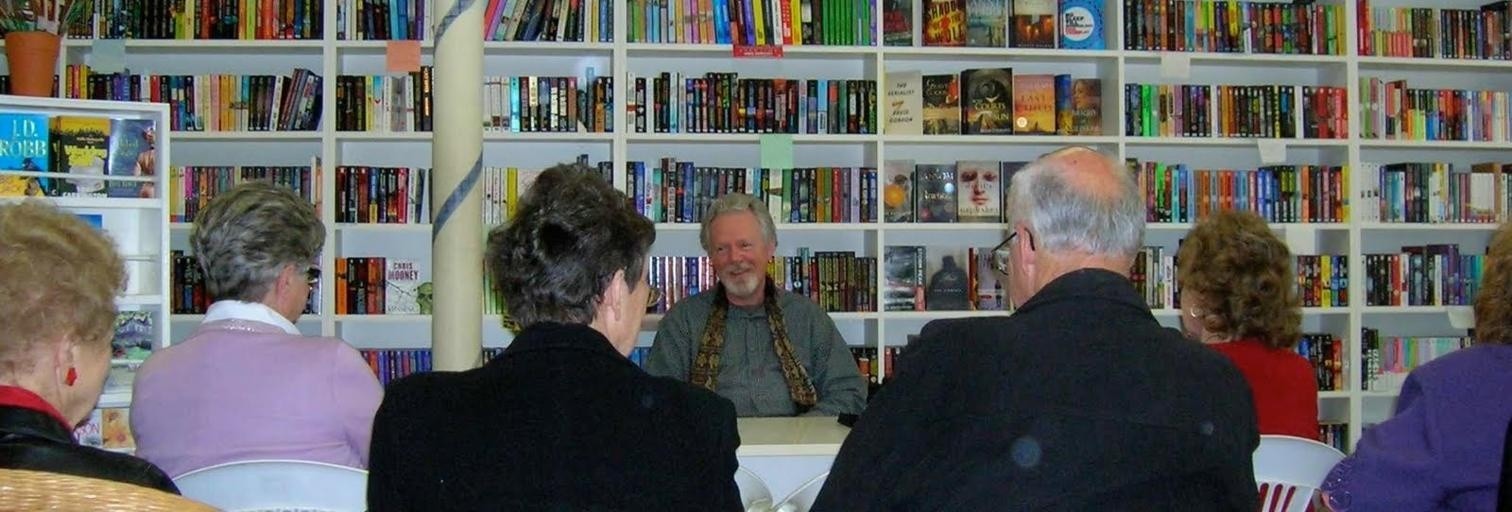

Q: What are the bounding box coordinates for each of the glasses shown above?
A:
[992,230,1035,275]
[621,266,664,307]
[304,263,320,284]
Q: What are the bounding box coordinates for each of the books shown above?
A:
[482,0,614,133]
[1356,0,1509,143]
[1123,1,1352,142]
[882,1,1106,137]
[335,163,434,389]
[1125,158,1348,391]
[883,156,1031,378]
[169,157,322,316]
[1313,420,1378,465]
[625,160,878,389]
[46,1,324,132]
[0,111,157,198]
[335,0,433,132]
[483,160,612,365]
[1359,326,1477,392]
[626,0,877,135]
[1359,158,1508,304]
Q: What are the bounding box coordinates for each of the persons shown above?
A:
[1303,219,1512,508]
[1175,206,1320,511]
[365,160,746,512]
[1,201,183,496]
[126,180,385,512]
[803,148,1266,508]
[642,195,872,418]
